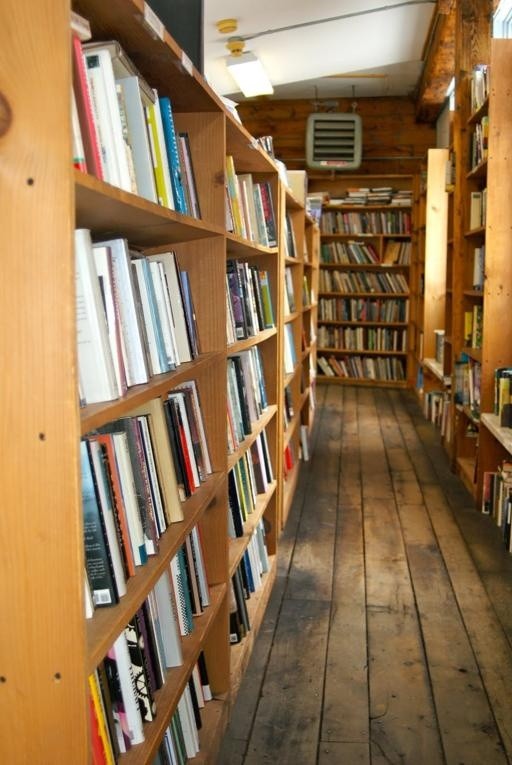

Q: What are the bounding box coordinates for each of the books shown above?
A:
[71,9,202,222]
[224,154,278,249]
[256,135,276,161]
[471,64,490,115]
[284,384,295,432]
[320,269,410,293]
[284,323,298,373]
[493,367,512,429]
[422,391,451,443]
[228,427,273,539]
[79,380,212,619]
[308,187,415,206]
[284,438,295,480]
[286,210,297,258]
[318,325,407,352]
[463,187,485,231]
[304,235,309,263]
[469,116,488,172]
[302,275,310,306]
[434,330,452,376]
[88,520,211,765]
[454,352,480,417]
[320,297,410,323]
[298,425,309,462]
[218,260,274,344]
[317,354,405,380]
[415,366,424,389]
[150,648,213,765]
[463,305,483,350]
[227,345,268,454]
[284,266,296,316]
[75,227,200,409]
[227,516,270,646]
[311,289,316,305]
[310,353,317,410]
[320,210,410,234]
[472,244,484,293]
[302,323,317,352]
[415,329,423,362]
[305,195,322,227]
[320,239,412,265]
[481,459,512,553]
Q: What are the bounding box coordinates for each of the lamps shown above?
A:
[225,35,246,57]
[215,17,237,34]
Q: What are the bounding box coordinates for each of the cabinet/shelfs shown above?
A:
[0,0,321,764]
[305,173,417,389]
[413,38,512,533]
